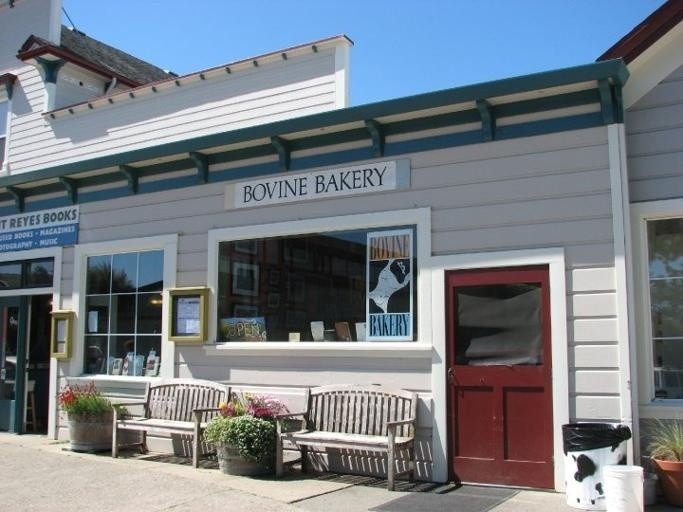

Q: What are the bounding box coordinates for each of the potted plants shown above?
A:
[638,414,682,508]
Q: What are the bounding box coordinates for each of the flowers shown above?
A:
[56,379,115,416]
[202,389,290,465]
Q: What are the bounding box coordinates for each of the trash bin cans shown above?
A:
[562,423,618,511]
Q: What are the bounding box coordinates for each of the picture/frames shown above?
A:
[230,240,259,320]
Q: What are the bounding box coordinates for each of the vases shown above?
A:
[214,443,268,475]
[69,413,114,451]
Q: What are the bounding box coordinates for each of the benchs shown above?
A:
[110,378,233,469]
[269,382,420,490]
